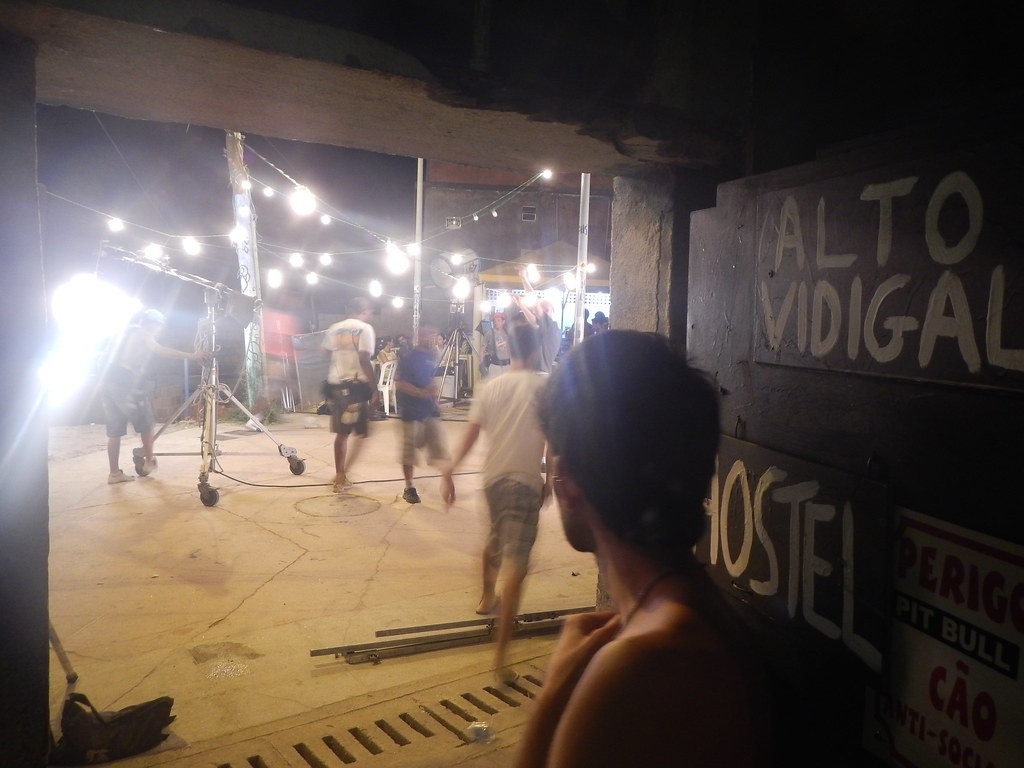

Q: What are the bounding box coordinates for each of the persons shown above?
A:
[517,327,772,768]
[90,300,610,684]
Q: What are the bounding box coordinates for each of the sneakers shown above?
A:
[403,488,421,504]
[333,475,353,493]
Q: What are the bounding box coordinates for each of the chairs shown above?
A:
[376,360,397,416]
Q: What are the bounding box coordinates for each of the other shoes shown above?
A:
[143,457,157,473]
[107,470,136,484]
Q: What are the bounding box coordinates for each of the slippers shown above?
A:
[490,667,519,687]
[475,593,500,615]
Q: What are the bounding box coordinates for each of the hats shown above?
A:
[491,312,505,321]
[346,296,369,314]
[143,309,166,324]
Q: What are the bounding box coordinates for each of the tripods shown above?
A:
[434,305,487,409]
